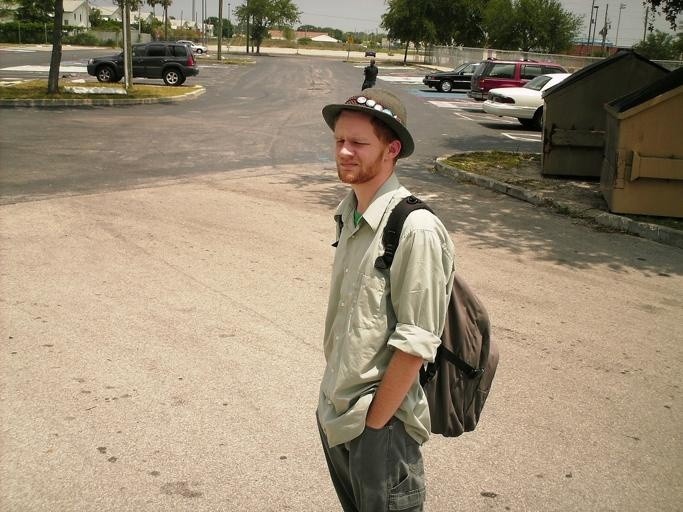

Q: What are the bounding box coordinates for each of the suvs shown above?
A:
[86,42,198,87]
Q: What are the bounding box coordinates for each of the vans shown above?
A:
[466,57,568,102]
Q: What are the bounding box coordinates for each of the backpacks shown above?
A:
[339,196,500,436]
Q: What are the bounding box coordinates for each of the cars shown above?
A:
[482,73,573,128]
[175,40,207,54]
[422,62,480,93]
[365,49,375,58]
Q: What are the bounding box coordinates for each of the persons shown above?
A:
[314,87,460,512]
[360,60,377,92]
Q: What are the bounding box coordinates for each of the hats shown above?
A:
[322,89,414,158]
